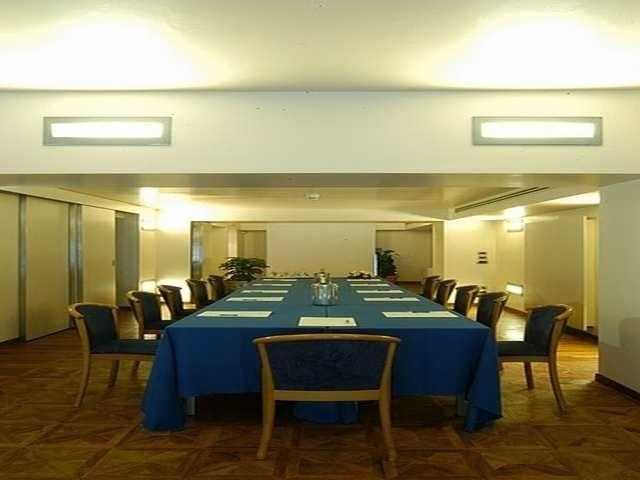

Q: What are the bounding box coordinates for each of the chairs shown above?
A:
[251,332,402,462]
[65,275,226,408]
[420,274,573,410]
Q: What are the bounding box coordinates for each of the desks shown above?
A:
[137,275,501,433]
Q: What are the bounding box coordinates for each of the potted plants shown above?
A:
[216,256,271,291]
[375,247,400,284]
